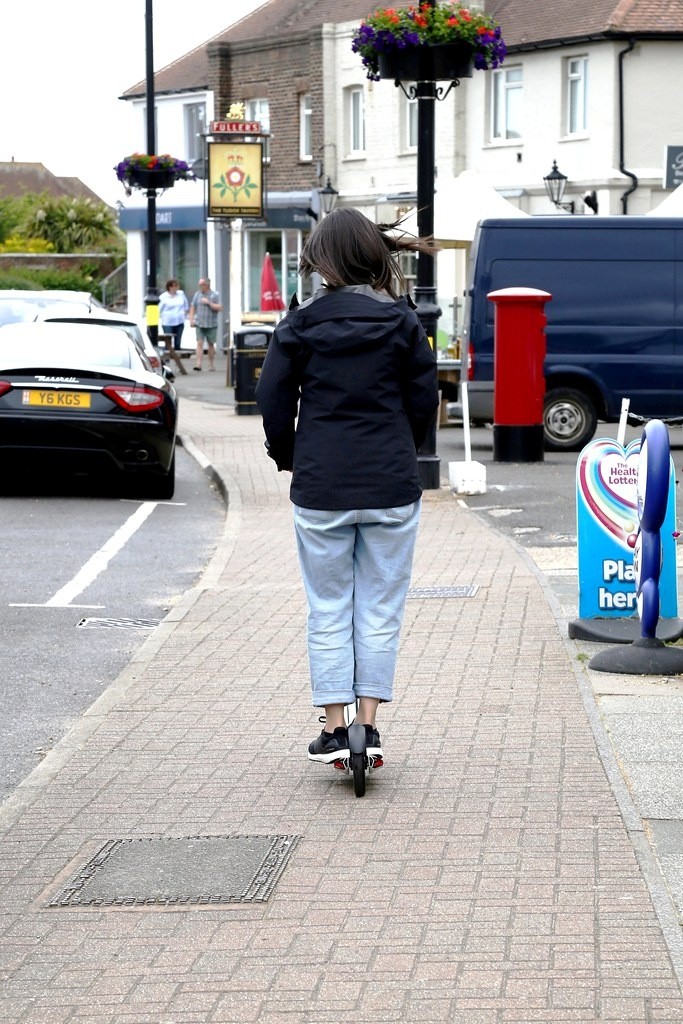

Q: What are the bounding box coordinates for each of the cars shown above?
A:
[0,288,165,380]
[0,323,181,500]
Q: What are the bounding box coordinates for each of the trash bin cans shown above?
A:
[233,325,276,415]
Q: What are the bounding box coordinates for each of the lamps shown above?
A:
[317,177,340,216]
[542,158,574,216]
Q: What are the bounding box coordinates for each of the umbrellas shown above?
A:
[259,252,286,321]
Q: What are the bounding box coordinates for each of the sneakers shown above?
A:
[306,716,351,763]
[363,723,386,767]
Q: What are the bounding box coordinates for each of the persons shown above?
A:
[189,277,223,371]
[254,208,441,765]
[158,279,189,350]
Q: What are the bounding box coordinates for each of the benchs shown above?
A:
[156,332,195,375]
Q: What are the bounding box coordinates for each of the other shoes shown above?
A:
[193,366,203,371]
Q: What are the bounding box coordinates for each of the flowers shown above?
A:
[114,151,198,186]
[349,3,518,83]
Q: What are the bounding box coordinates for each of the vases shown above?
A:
[375,35,476,79]
[136,166,175,189]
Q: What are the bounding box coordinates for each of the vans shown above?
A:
[459,215,683,454]
